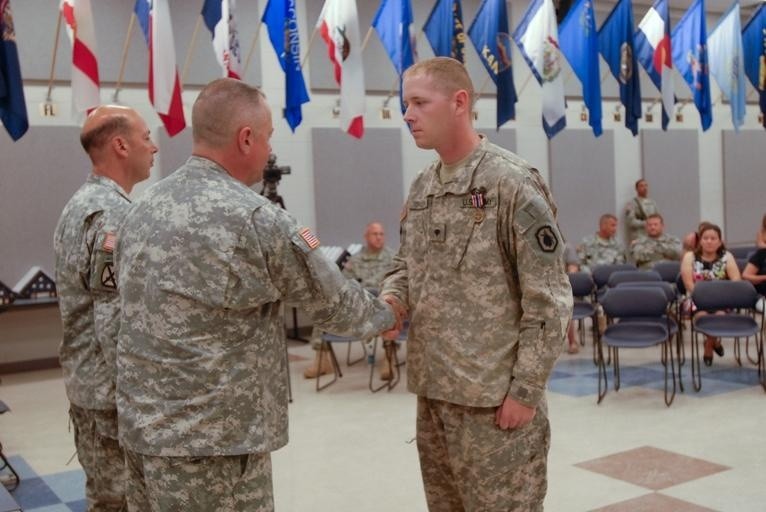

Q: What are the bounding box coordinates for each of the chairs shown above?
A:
[316,286,412,393]
[566,240,765,407]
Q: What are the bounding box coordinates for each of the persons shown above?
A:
[114,78,399,512]
[303,220,398,380]
[378,58,574,512]
[53,105,160,512]
[562,178,765,369]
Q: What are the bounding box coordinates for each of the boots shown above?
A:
[303,351,331,378]
[381,351,396,379]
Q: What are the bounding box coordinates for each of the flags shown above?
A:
[201,0,244,82]
[261,0,310,133]
[1,0,30,139]
[374,0,521,130]
[59,0,100,124]
[133,0,187,138]
[513,0,765,138]
[318,0,364,138]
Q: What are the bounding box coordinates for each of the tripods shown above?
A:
[263,193,312,343]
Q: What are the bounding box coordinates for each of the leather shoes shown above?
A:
[713,342,724,357]
[703,340,713,367]
